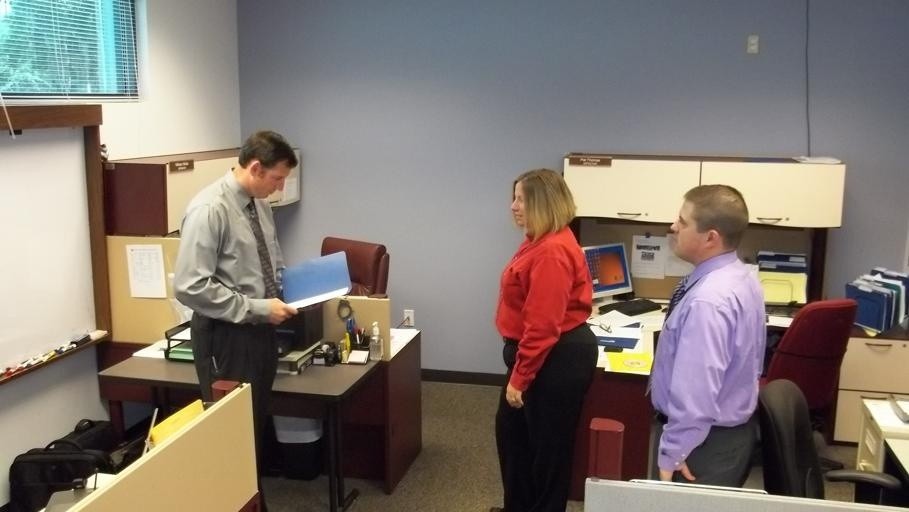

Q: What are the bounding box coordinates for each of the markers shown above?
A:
[0,344,76,375]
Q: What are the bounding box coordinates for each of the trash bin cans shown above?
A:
[273,415,328,480]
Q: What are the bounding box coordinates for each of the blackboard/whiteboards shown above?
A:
[1,128,96,375]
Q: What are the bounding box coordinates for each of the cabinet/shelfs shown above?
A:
[831,329,909,445]
[563,156,845,230]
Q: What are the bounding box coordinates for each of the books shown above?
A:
[590,308,641,329]
[280,249,353,313]
[844,266,909,333]
[589,325,642,350]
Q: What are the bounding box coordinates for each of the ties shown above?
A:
[247,200,280,300]
[645,273,690,397]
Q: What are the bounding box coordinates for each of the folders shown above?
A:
[845,268,908,334]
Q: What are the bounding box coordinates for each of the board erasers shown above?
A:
[70,333,91,348]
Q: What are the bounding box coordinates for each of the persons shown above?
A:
[493,165,600,511]
[172,127,299,512]
[641,181,771,490]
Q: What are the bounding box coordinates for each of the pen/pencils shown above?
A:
[349,327,370,346]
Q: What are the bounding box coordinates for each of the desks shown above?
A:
[97,329,429,510]
[567,298,805,500]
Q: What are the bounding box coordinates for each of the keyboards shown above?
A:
[599,298,662,317]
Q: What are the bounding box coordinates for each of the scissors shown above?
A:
[347,318,356,335]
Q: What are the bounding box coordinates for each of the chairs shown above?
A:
[320,237,391,298]
[760,298,898,502]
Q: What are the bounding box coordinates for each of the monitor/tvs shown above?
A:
[582,242,634,305]
